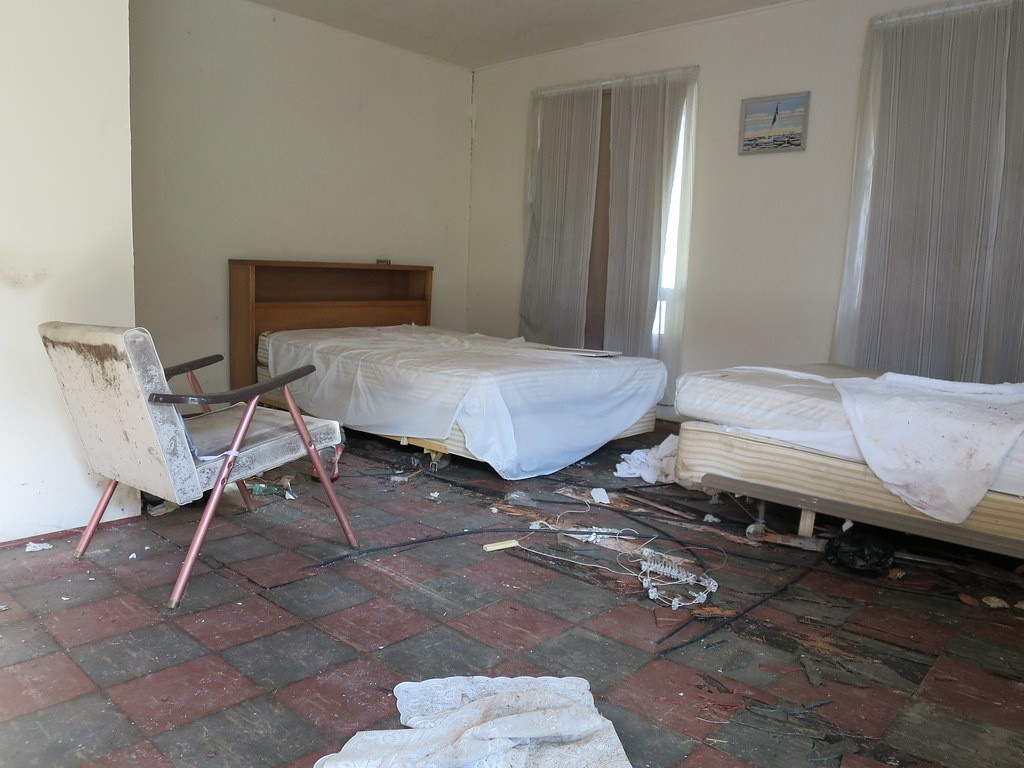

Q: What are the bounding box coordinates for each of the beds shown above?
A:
[223,256,669,479]
[671,364,1024,564]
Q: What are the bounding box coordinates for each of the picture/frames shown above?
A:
[739,90,810,153]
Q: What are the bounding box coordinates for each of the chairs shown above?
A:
[35,320,357,606]
